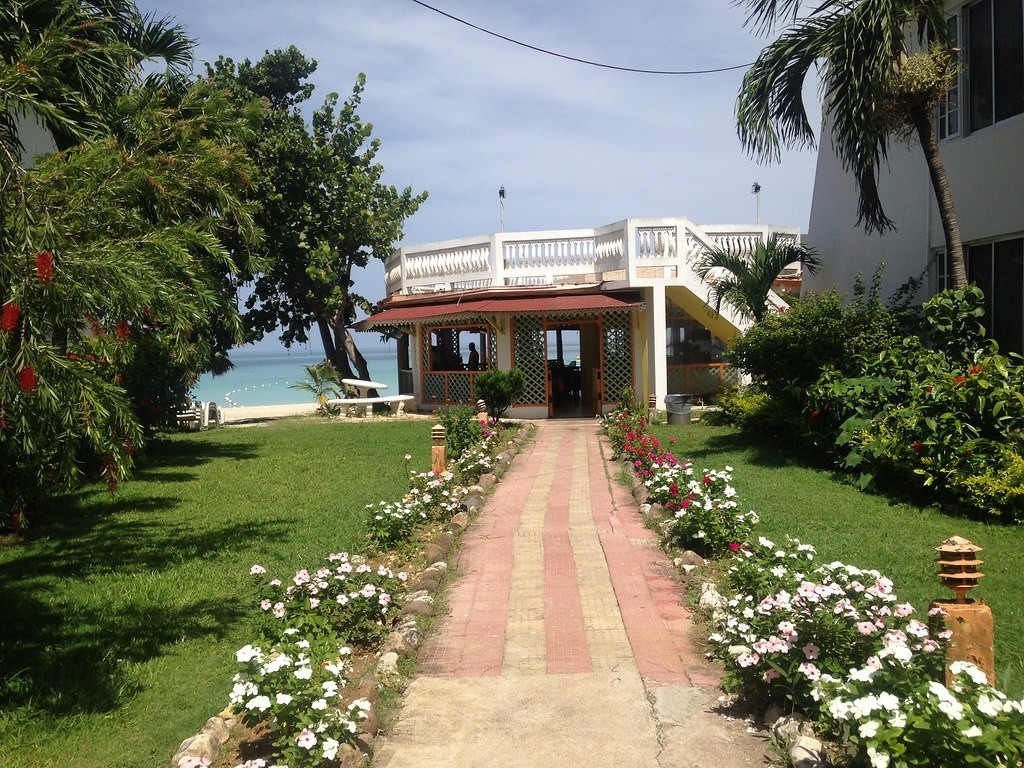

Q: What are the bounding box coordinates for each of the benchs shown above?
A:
[327,394,414,419]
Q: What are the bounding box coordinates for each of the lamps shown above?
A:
[649,394,657,407]
[477,399,487,412]
[934,534,985,603]
[431,424,447,445]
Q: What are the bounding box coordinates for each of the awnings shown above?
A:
[346,294,646,332]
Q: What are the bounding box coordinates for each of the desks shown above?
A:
[342,379,388,412]
[563,365,582,396]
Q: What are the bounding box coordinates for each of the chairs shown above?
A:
[548,360,580,401]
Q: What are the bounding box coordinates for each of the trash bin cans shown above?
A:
[664,393,693,426]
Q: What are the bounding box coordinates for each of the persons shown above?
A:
[689,333,714,364]
[466,341,479,370]
